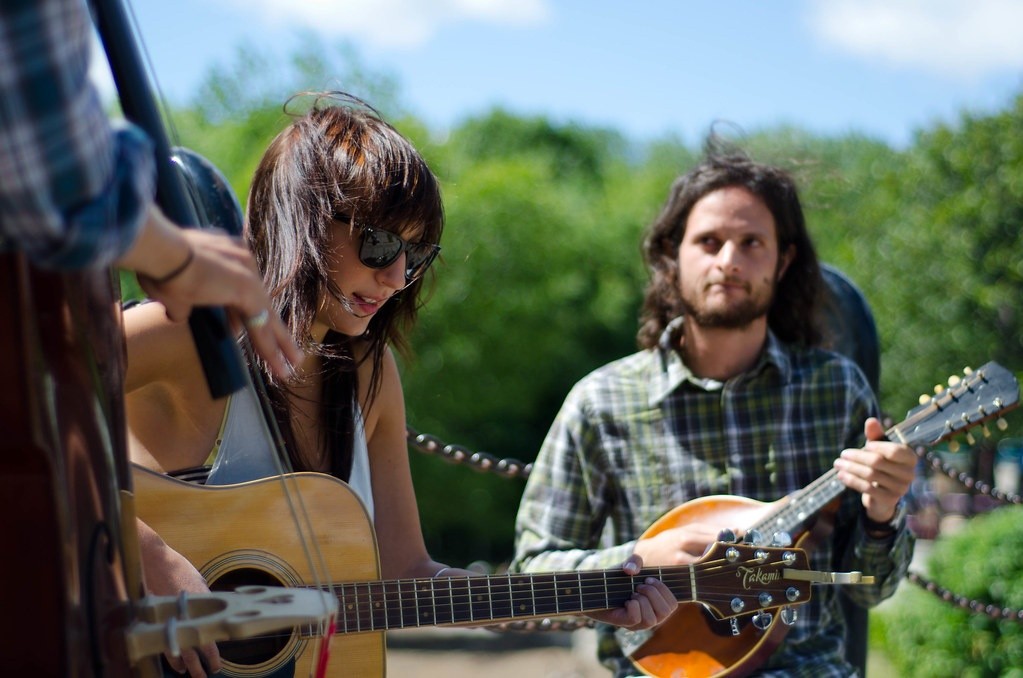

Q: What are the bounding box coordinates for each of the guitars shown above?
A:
[612,358,1023,678]
[113,458,877,678]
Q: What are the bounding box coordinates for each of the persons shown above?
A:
[507,118,917,678]
[124,91,677,678]
[0,0,303,678]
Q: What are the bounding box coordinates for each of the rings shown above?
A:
[247,309,269,327]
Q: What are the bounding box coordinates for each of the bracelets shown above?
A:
[435,567,449,577]
[148,246,193,284]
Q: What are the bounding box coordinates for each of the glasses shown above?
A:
[332,212,442,280]
